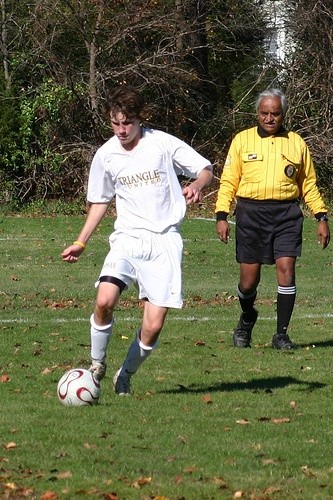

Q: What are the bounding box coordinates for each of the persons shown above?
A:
[215,89,331,349]
[60,86,213,395]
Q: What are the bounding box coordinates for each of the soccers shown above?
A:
[57,368,101,407]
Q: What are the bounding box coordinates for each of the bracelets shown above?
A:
[73,241,86,249]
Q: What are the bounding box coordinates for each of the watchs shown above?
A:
[317,215,329,222]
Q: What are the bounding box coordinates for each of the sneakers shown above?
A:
[233,311,257,348]
[272,334,298,354]
[88,365,106,382]
[112,373,130,397]
